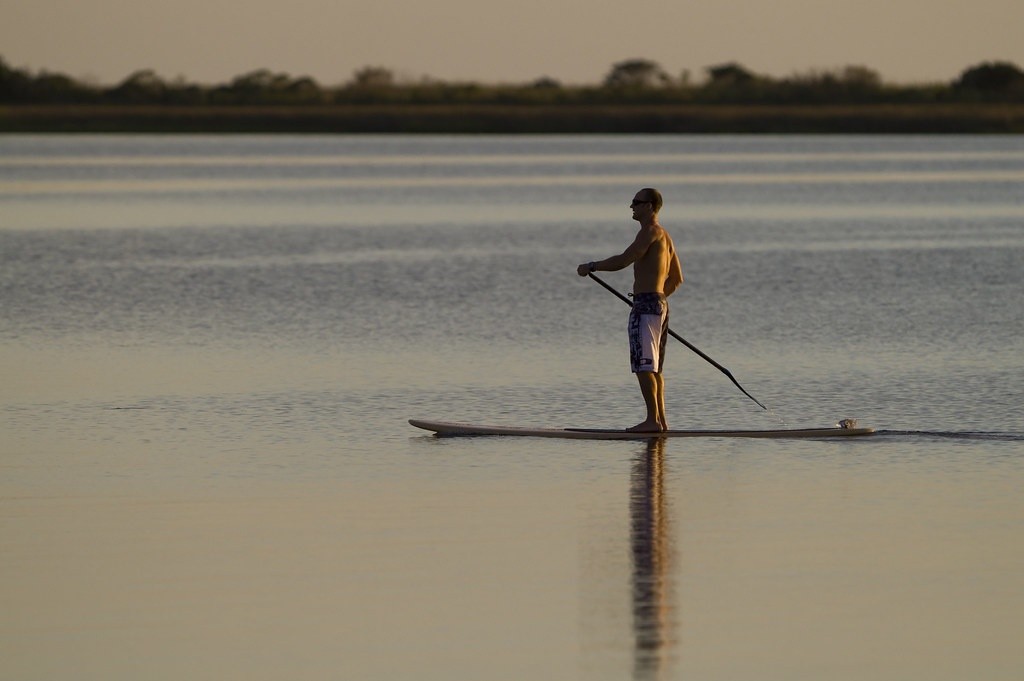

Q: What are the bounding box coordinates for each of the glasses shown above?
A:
[632,198,646,205]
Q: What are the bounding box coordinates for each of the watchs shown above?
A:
[589,262,596,272]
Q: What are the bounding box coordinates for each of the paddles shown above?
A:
[585,270,766,410]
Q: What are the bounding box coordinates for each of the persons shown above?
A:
[576,187,685,434]
